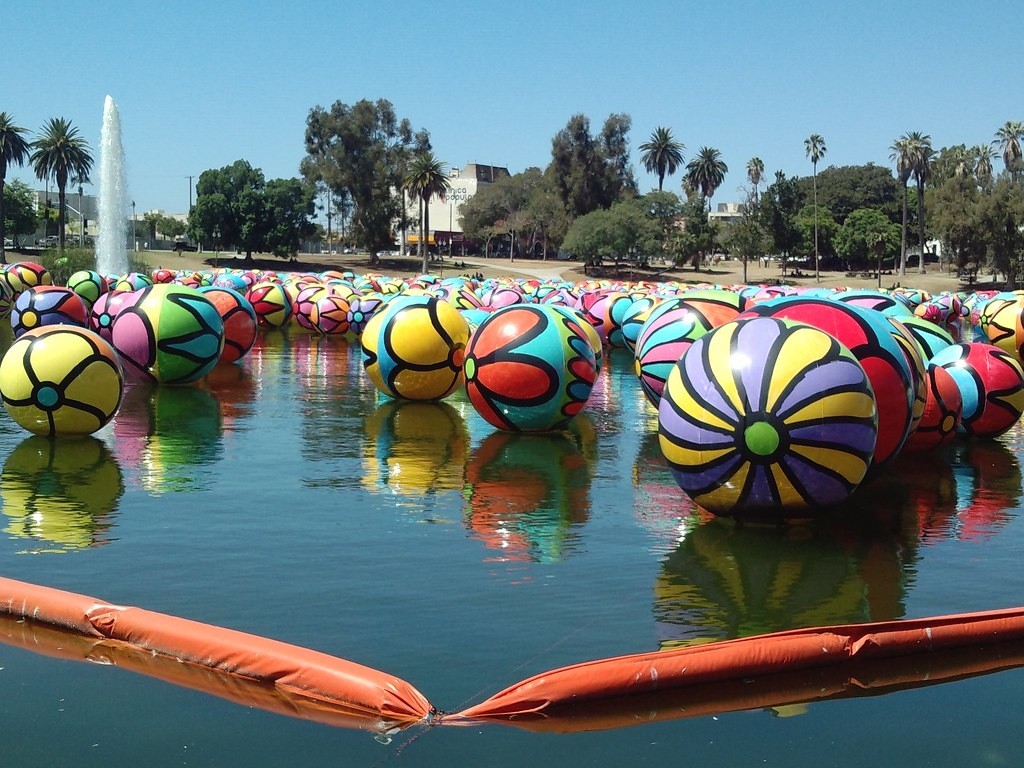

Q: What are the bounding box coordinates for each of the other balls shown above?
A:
[0,263,1024,526]
